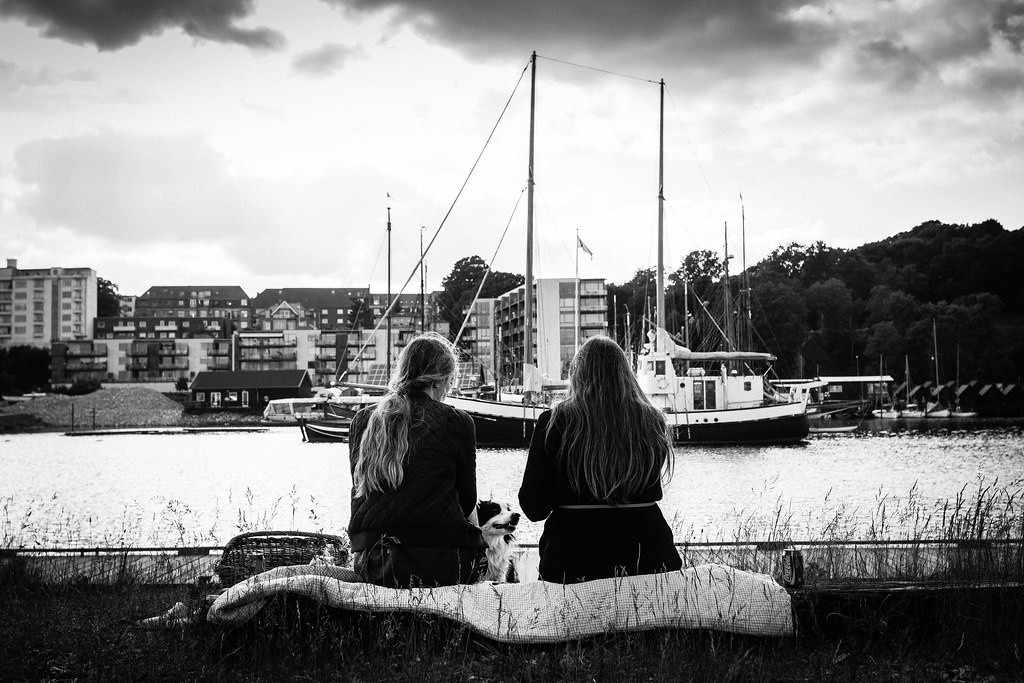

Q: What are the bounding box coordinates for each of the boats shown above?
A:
[763,374,894,419]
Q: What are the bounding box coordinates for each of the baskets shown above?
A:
[214,531,348,589]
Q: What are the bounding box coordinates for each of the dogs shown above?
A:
[477,499,522,583]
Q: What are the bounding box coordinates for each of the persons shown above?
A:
[518,336,683,584]
[347,332,488,590]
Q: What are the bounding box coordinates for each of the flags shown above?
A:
[578,236,594,260]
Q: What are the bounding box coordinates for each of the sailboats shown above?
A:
[300,50,811,448]
[872,318,976,419]
